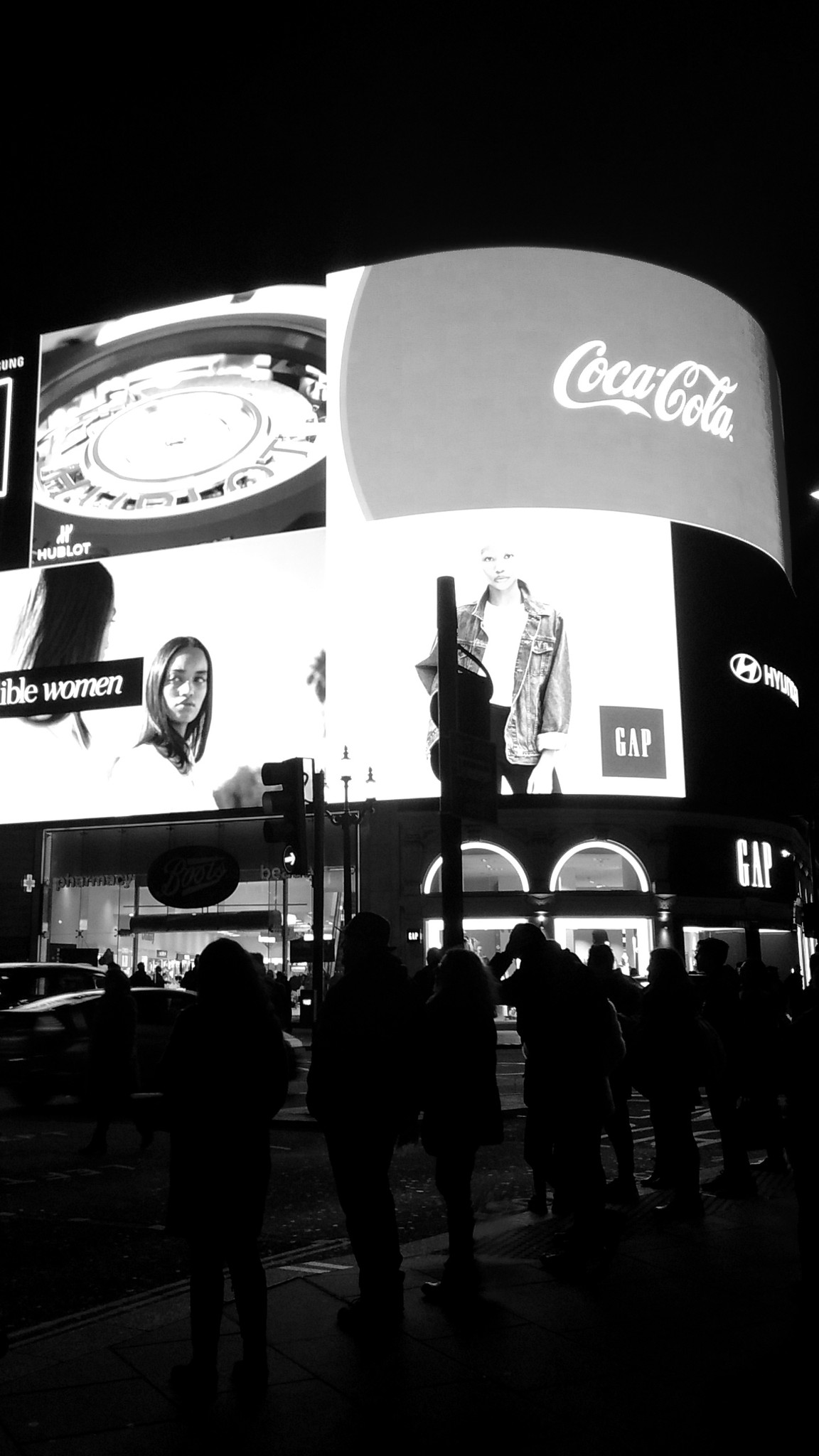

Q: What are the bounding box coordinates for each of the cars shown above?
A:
[3,989,304,1121]
[0,962,110,1015]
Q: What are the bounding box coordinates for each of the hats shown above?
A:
[334,911,397,952]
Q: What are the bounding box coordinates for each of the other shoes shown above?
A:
[75,1136,108,1156]
[170,1362,218,1395]
[233,1358,271,1394]
[419,1157,819,1346]
[136,1111,154,1150]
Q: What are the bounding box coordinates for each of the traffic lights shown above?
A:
[258,757,313,879]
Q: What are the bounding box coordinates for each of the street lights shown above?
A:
[316,746,378,946]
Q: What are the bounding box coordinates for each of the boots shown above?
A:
[384,1269,407,1323]
[336,1292,384,1340]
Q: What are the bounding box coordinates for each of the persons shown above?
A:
[122,636,224,812]
[1,562,115,819]
[128,960,171,988]
[306,912,819,1287]
[414,532,574,794]
[166,936,313,1369]
